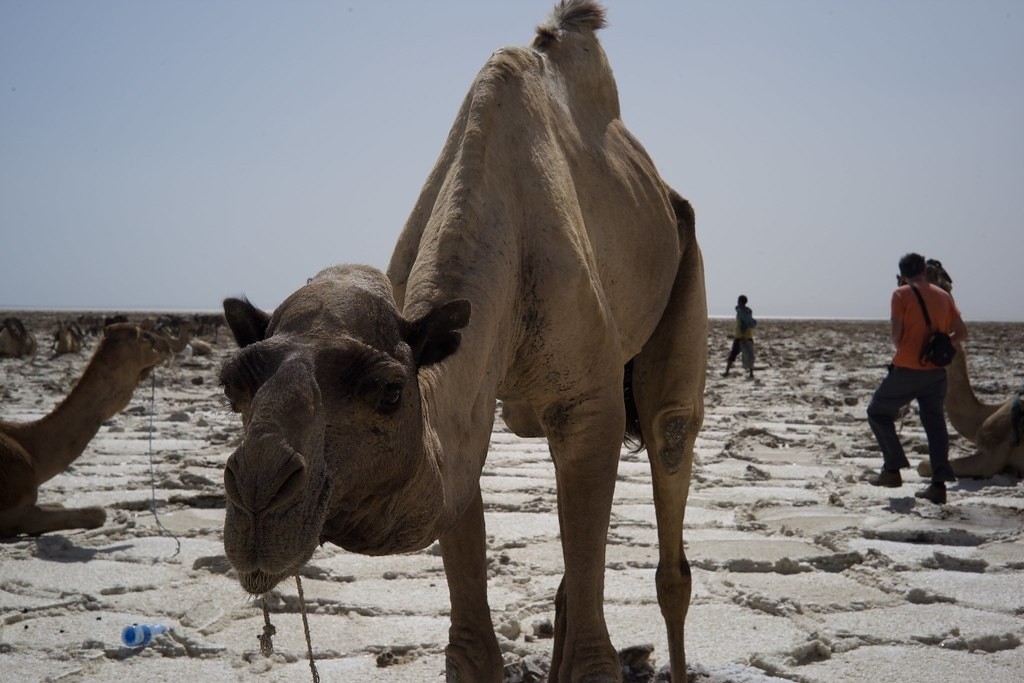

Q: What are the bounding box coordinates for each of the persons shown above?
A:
[866,253,969,507]
[719,295,756,379]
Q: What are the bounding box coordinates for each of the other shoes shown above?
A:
[915,485,947,504]
[720,373,728,377]
[869,470,902,487]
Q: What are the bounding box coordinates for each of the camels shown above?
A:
[217,0,707,683]
[917,342,1024,477]
[0,307,230,545]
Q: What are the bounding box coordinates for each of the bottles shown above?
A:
[121,624,169,647]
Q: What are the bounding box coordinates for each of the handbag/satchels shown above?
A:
[918,331,956,367]
